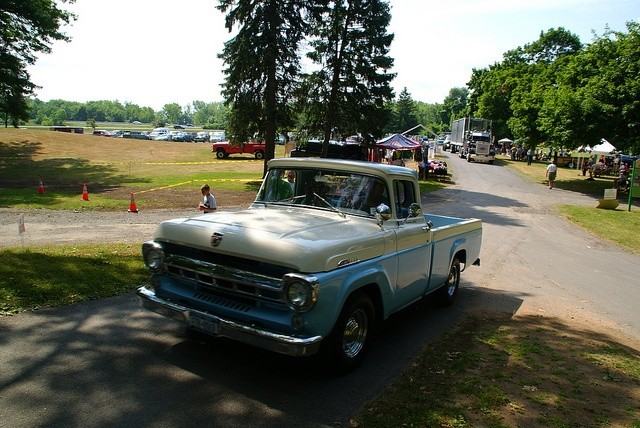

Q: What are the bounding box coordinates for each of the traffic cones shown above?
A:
[82,184,90,201]
[38,180,44,193]
[128,192,138,212]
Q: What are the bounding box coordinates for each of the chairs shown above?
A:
[354,181,390,215]
[301,182,331,207]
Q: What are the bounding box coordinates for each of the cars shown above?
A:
[210,132,225,143]
[174,125,184,128]
[443,136,450,151]
[156,134,168,140]
[169,131,193,142]
[437,136,444,144]
[194,132,210,143]
[274,134,286,144]
[93,130,150,139]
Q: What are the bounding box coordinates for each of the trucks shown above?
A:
[450,117,495,164]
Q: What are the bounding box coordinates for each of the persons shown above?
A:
[581,159,587,177]
[534,148,547,161]
[526,147,533,166]
[546,163,557,189]
[270,169,293,202]
[619,162,632,185]
[588,153,596,181]
[559,148,565,157]
[612,154,619,176]
[285,169,296,196]
[198,182,217,214]
[547,146,554,159]
[517,146,526,161]
[510,145,516,161]
[597,154,606,166]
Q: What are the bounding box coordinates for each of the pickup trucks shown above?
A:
[136,157,482,361]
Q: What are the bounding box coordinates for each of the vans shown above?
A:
[149,128,168,140]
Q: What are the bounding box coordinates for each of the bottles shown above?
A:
[199,202,204,213]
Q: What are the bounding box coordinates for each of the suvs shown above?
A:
[212,136,265,159]
